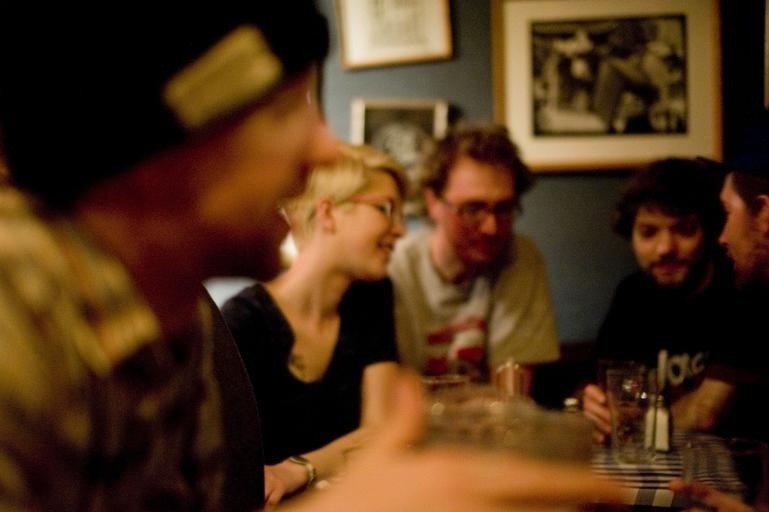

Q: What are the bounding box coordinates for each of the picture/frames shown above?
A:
[340,93,456,217]
[334,1,456,72]
[485,3,726,174]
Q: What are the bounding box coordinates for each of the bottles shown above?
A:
[643,394,671,451]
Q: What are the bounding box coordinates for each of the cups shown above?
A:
[608,363,657,466]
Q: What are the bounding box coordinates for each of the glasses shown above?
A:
[438,197,523,225]
[346,194,394,219]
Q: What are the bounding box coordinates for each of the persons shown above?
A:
[681,145,769,505]
[221,144,429,509]
[573,153,731,446]
[592,23,687,132]
[388,128,561,400]
[0,0,627,511]
[669,477,767,511]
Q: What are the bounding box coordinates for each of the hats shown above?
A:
[4,2,329,210]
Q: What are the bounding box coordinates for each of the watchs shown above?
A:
[288,454,318,485]
